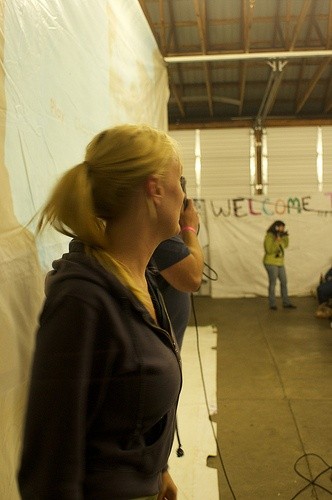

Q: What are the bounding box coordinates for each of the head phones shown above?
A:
[276,226,279,230]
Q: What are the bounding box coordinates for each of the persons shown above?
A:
[20,124,185,500]
[146,177,203,351]
[264,221,297,308]
[317,268,332,304]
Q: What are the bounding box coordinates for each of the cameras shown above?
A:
[280,231,288,237]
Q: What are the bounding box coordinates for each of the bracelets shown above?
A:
[183,226,197,233]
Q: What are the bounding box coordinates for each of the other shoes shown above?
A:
[270,306,277,310]
[283,304,297,309]
